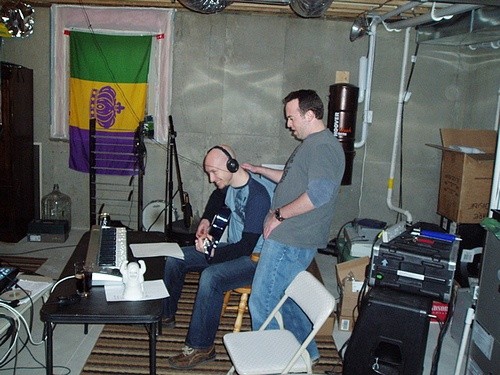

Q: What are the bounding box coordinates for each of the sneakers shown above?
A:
[162,314,176,329]
[168,341,215,369]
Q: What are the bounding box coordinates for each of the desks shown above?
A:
[0,274,53,375]
[40,231,164,375]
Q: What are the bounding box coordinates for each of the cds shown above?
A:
[0,290,28,301]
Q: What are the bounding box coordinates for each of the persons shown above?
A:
[161,145,270,369]
[120,260,146,299]
[241,90,345,365]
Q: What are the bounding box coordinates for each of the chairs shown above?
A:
[221,253,259,331]
[223,271,336,375]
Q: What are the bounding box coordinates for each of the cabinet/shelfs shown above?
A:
[0,61,34,243]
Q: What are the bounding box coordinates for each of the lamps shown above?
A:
[350,11,370,42]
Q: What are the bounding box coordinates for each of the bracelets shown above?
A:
[275,209,286,221]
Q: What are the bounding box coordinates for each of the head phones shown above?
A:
[208,145,239,172]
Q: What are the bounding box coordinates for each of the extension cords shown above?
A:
[382,220,407,243]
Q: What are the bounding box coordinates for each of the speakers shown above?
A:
[343,282,432,375]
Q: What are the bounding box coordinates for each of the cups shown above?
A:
[100,213,110,226]
[74,261,92,297]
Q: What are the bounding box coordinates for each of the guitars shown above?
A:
[204,204,233,260]
[168,115,194,231]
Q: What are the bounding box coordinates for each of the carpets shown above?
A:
[79,258,344,375]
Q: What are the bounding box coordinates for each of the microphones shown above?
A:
[184,194,192,228]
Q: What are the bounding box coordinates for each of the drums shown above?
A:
[142,198,177,232]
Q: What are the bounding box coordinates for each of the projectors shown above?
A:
[343,224,382,259]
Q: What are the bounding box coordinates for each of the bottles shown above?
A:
[42,185,71,232]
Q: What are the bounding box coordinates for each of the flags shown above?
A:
[68,31,150,175]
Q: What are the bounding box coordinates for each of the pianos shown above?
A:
[84,224,127,275]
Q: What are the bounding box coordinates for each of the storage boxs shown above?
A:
[27,218,69,242]
[425,128,497,224]
[334,256,370,331]
